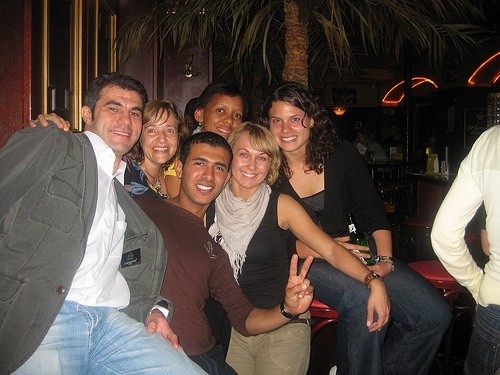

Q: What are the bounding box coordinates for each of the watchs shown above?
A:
[375,255,395,271]
[280,300,300,319]
[364,271,380,285]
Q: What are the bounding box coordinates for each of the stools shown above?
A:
[399,221,433,261]
[306,299,342,375]
[407,261,476,375]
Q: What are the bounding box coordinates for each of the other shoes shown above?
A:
[329,366,338,375]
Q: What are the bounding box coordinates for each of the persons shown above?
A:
[161,77,245,199]
[1,71,209,375]
[430,124,499,375]
[353,124,444,173]
[126,129,314,375]
[203,121,391,374]
[122,99,192,197]
[258,80,451,374]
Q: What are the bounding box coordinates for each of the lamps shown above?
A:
[332,89,346,116]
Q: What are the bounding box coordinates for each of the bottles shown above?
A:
[346,214,376,265]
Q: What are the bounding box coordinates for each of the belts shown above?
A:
[290,317,316,325]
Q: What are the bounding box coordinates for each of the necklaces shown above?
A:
[139,161,163,191]
